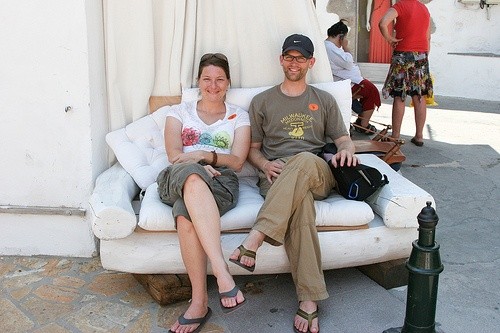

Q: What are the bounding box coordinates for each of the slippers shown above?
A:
[229,244,256,272]
[219,285,247,314]
[293,303,319,333]
[168,305,212,333]
[412,138,423,146]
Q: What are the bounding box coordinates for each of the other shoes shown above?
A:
[355,121,376,134]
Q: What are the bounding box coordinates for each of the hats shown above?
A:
[282,34,314,58]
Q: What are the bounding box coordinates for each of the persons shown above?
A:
[229,34,361,333]
[156,53,251,333]
[324,21,381,134]
[380,0,433,147]
[366,0,396,63]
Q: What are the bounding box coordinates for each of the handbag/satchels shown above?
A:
[322,145,388,200]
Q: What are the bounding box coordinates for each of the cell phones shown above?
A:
[340,34,344,40]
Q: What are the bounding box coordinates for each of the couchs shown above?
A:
[91,96,435,278]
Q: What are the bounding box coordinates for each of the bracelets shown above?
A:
[211,151,217,166]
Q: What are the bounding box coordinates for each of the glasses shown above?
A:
[201,54,227,62]
[283,54,307,63]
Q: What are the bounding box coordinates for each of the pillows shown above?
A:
[182,80,356,138]
[107,103,182,189]
